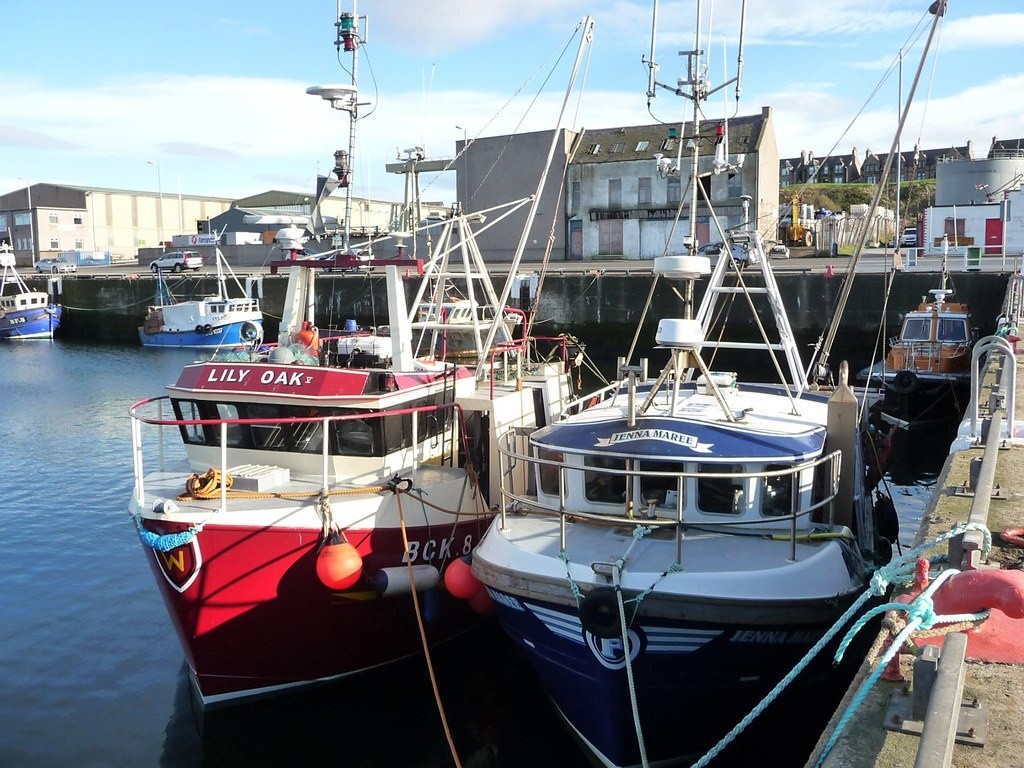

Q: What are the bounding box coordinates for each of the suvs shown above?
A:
[899,227,916,248]
[149,250,203,273]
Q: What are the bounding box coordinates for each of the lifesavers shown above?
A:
[875,535,893,561]
[878,495,898,544]
[578,587,624,639]
[196,323,213,334]
[892,369,919,395]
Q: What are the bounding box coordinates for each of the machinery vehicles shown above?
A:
[784,190,815,247]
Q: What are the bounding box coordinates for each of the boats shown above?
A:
[0,226,62,340]
[854,234,983,415]
[137,223,266,349]
[129,0,949,767]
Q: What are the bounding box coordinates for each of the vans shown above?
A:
[322,248,375,272]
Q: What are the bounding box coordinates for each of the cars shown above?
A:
[34,257,78,274]
[285,247,328,261]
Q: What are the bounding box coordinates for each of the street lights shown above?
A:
[145,160,166,255]
